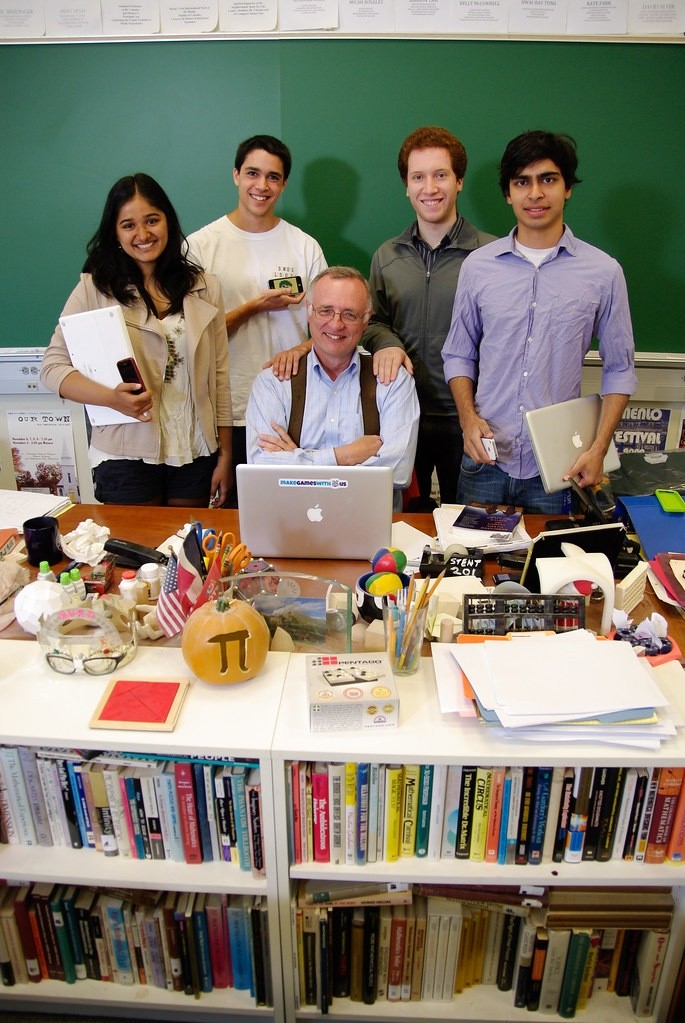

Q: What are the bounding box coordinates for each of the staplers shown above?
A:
[492,553,527,584]
[103,538,169,570]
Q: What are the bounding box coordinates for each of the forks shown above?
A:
[397,588,409,612]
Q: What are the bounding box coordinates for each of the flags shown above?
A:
[155,529,223,639]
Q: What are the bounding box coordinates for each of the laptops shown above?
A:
[523,393,621,493]
[236,464,392,561]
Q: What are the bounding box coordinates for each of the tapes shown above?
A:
[431,544,469,562]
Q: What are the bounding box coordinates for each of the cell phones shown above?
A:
[269,276,304,294]
[117,358,147,395]
[481,438,498,461]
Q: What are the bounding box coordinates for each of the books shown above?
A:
[0,744,264,881]
[285,759,685,866]
[290,878,675,1018]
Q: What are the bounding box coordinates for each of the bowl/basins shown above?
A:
[356,572,416,624]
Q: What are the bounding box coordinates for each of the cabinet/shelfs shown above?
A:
[1,637,684,1023]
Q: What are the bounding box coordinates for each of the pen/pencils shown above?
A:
[212,543,222,565]
[219,545,231,565]
[217,530,223,545]
[388,568,448,672]
[221,557,231,578]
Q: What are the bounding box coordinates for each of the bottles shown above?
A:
[70,568,86,600]
[118,571,138,601]
[140,563,161,600]
[59,572,78,606]
[37,561,57,583]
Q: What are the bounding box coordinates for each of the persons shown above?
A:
[180,135,330,509]
[243,265,420,513]
[442,131,635,518]
[38,173,231,508]
[357,126,501,511]
[0,882,273,1007]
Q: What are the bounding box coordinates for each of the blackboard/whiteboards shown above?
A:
[0,33,685,370]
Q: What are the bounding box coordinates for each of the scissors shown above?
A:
[190,522,252,576]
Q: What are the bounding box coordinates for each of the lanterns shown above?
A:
[182,597,269,686]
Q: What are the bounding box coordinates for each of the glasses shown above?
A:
[44,651,128,677]
[312,305,366,326]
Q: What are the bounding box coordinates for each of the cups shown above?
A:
[383,589,429,676]
[23,517,63,567]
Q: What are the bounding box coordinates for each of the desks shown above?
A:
[0,503,684,660]
